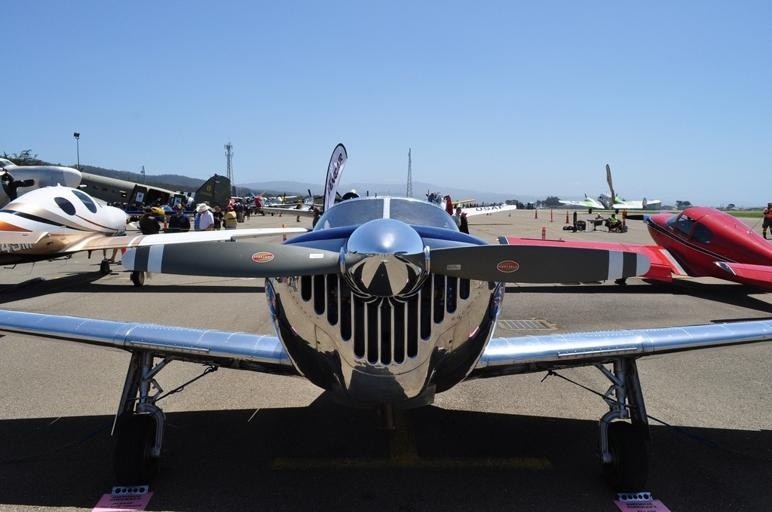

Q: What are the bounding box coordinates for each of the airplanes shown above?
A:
[0,183,772,512]
[79,167,235,222]
[227,186,523,221]
[0,155,87,202]
[557,189,665,215]
[0,181,134,276]
[485,201,772,291]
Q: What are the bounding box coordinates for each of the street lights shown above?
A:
[73,132,82,170]
[139,164,147,184]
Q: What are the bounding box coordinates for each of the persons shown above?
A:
[428,193,437,202]
[592,214,604,231]
[124,188,318,236]
[623,208,628,219]
[609,213,621,231]
[460,213,469,234]
[763,202,772,239]
[452,208,461,228]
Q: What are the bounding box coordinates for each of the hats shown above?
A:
[195,203,210,213]
